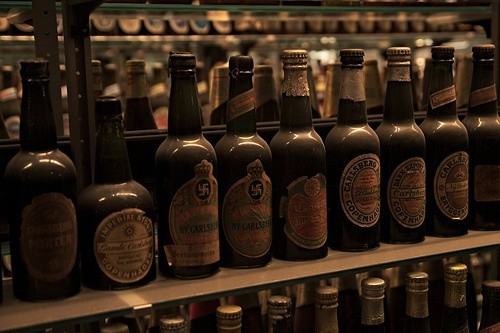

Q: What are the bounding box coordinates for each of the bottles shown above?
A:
[0,44,500,333]
